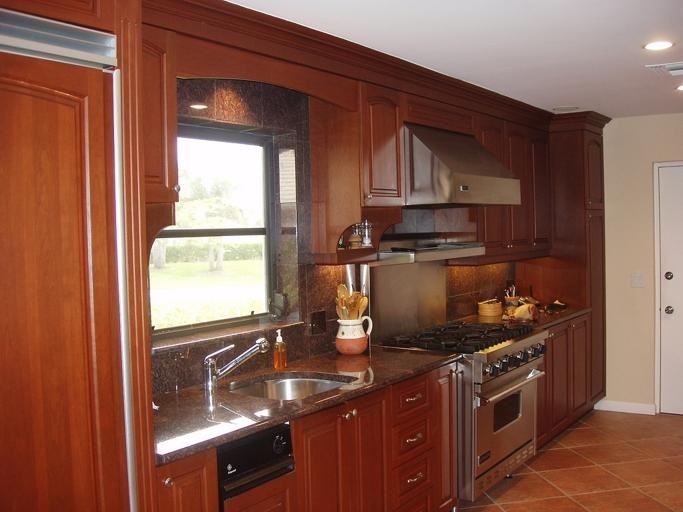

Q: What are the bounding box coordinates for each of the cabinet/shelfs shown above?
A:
[308,28,407,267]
[536,319,571,450]
[0,0,156,511]
[390,368,433,512]
[153,447,219,512]
[571,310,591,424]
[526,104,553,258]
[432,362,456,512]
[289,385,389,512]
[443,75,527,267]
[223,469,296,512]
[140,1,179,204]
[516,111,612,448]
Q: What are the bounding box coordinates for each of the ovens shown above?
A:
[471,329,550,480]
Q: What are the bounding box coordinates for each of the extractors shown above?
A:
[403,120,522,210]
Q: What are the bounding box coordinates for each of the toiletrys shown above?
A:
[273,328,287,370]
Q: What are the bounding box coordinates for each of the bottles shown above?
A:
[348,223,373,248]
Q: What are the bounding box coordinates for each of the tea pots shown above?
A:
[335,315,373,356]
[335,354,375,391]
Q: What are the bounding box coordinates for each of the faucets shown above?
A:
[204,338,271,394]
[204,394,273,425]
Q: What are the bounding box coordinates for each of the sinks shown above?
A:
[220,370,358,401]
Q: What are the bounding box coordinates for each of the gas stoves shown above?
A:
[373,319,533,355]
